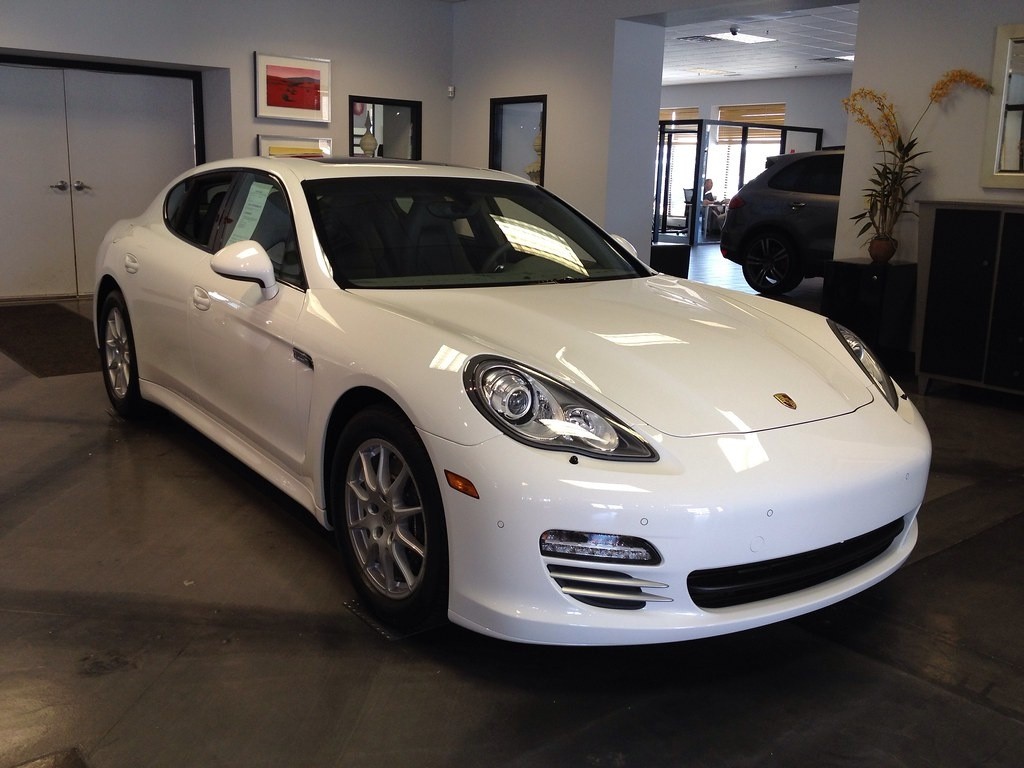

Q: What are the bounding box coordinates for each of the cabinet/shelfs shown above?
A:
[912,199,1024,397]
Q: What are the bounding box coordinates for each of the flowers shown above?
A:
[840,69,994,237]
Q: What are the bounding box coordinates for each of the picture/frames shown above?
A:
[256,133,333,156]
[353,103,375,137]
[253,50,331,124]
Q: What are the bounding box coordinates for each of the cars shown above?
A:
[90,158,933,647]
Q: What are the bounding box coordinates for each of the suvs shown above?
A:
[720,150,844,293]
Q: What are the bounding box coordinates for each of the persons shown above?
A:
[690,179,715,204]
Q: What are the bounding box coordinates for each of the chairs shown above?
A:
[324,204,377,279]
[676,188,694,235]
[399,194,462,274]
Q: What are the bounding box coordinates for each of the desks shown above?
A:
[686,203,729,240]
[820,258,918,377]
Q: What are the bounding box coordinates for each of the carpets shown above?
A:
[657,235,687,243]
[0,303,103,378]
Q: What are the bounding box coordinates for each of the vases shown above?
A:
[868,239,899,262]
